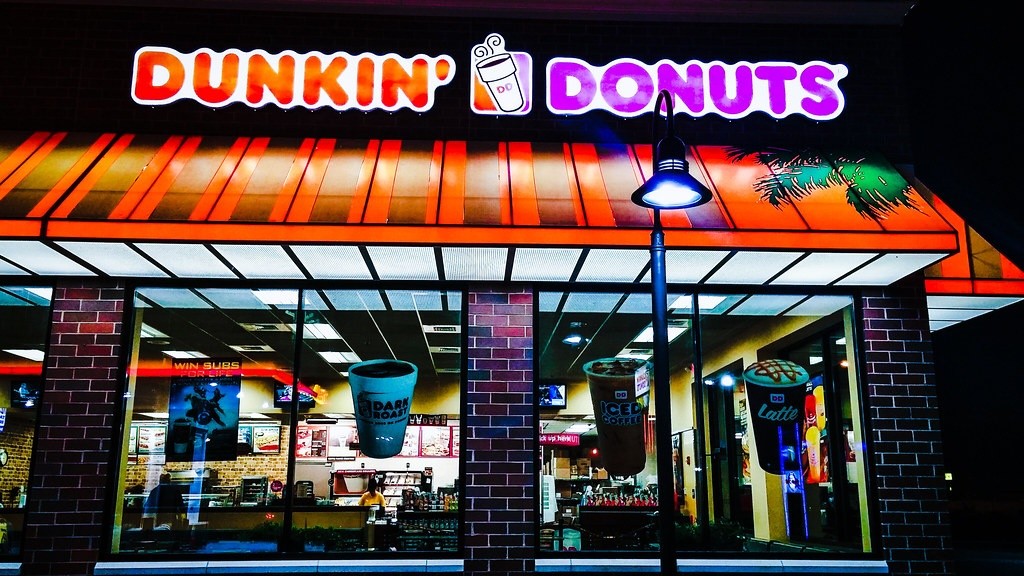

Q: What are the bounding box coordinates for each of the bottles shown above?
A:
[406,491,460,512]
[397,518,460,532]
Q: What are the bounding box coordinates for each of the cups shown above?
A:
[742,358,808,476]
[347,358,418,459]
[583,357,649,475]
[804,384,828,478]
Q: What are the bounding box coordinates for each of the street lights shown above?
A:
[630,88,714,576]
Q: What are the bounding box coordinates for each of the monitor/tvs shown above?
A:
[538,382,567,409]
[11,376,43,409]
[274,380,315,409]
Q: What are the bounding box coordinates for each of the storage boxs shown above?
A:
[545,457,592,498]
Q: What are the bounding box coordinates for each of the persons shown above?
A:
[143,474,185,509]
[358,478,386,508]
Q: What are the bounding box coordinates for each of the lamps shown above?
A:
[705,371,729,385]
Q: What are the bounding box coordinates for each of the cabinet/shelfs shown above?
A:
[240,475,268,502]
[551,450,610,502]
